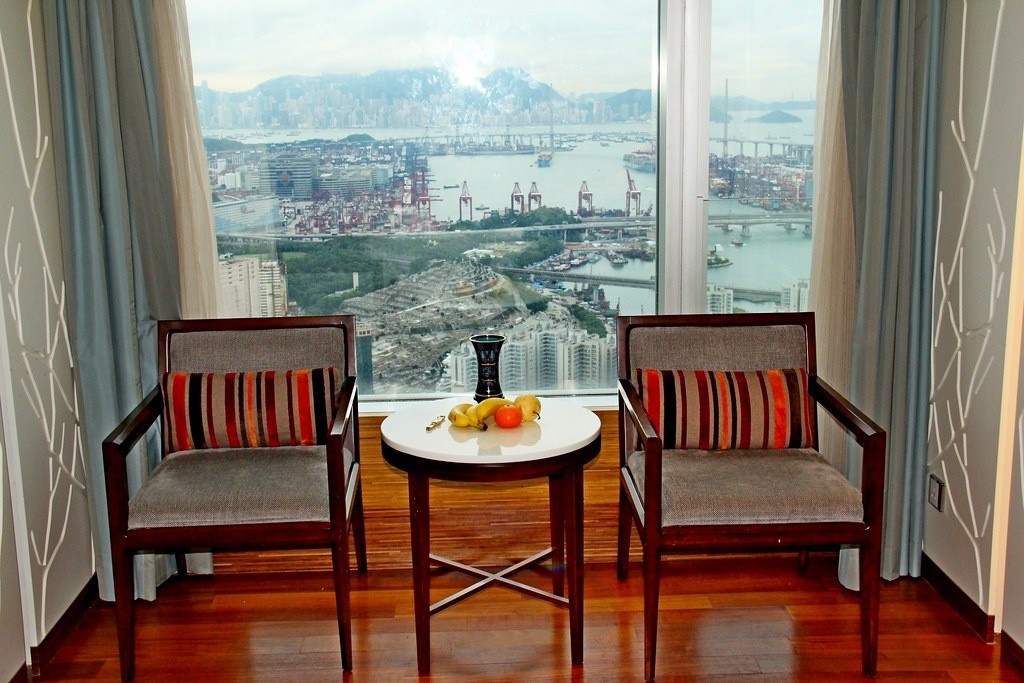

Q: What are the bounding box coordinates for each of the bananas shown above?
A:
[447,397,514,431]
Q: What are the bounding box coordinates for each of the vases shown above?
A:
[469,334,506,402]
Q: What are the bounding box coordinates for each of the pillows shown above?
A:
[633,367,814,451]
[159,367,341,460]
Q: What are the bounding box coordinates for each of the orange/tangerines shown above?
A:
[495,404,522,428]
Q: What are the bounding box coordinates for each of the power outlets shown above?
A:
[928,474,946,512]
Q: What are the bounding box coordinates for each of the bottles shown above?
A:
[469,334,506,404]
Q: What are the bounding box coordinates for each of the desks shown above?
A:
[380,393,602,674]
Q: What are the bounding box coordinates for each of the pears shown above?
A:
[513,394,541,421]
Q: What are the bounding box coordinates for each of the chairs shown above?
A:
[102,314,367,683]
[615,312,886,681]
